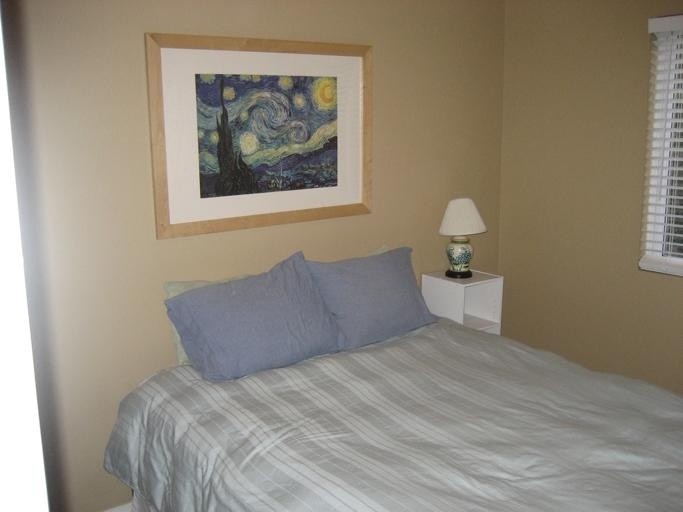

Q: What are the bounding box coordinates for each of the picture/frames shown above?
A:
[143,30,371,239]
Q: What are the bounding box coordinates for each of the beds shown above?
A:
[134,317,683,512]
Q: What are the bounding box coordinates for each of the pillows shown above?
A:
[160,245,436,383]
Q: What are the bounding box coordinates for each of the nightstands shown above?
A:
[420,268,503,337]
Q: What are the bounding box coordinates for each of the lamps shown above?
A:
[437,198,487,278]
